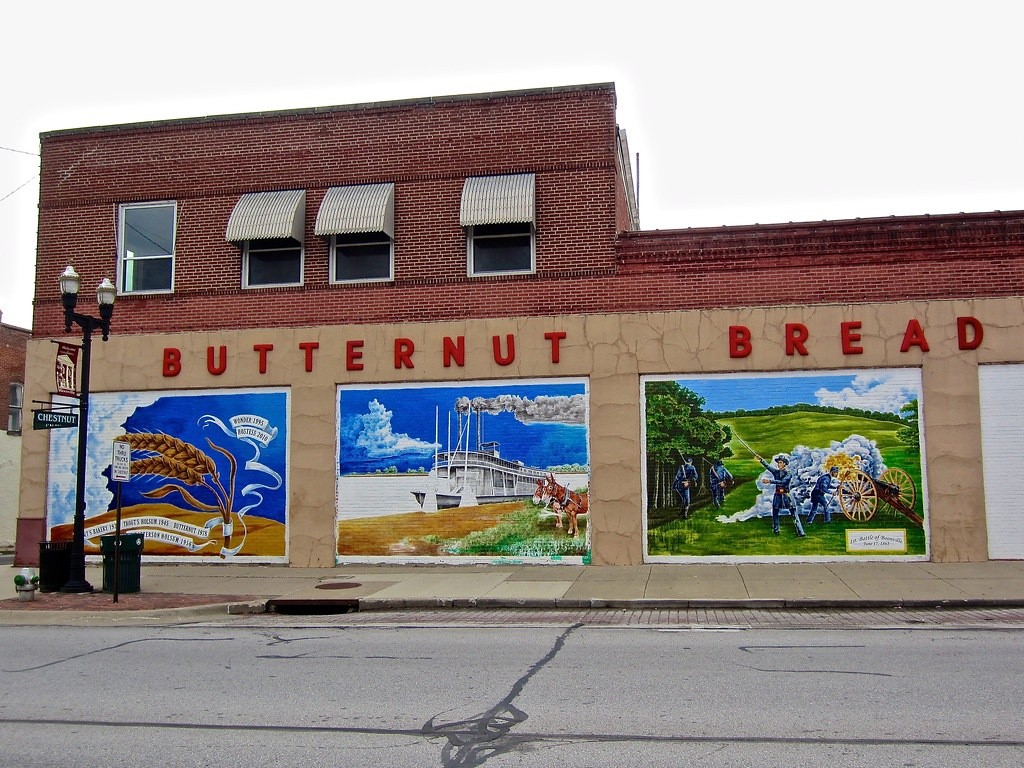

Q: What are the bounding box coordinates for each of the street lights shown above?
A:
[52,264,123,592]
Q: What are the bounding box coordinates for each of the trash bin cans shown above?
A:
[37,538,74,593]
[99,532,145,594]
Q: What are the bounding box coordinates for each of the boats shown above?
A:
[409,403,554,510]
